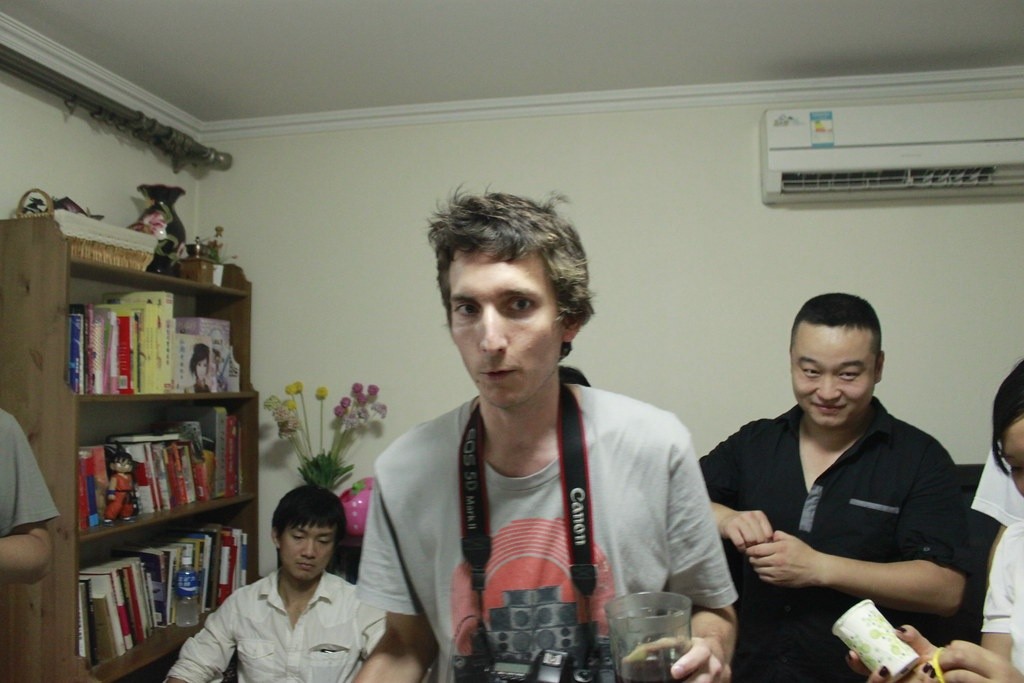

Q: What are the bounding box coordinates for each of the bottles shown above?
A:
[136,184,186,275]
[174,556,200,627]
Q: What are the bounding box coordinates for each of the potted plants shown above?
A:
[203,226,236,285]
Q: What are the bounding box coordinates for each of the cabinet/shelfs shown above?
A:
[0,215,260,682]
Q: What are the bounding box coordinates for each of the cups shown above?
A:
[832,599,920,683]
[604,590,692,683]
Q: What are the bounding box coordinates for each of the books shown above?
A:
[69,290,241,395]
[78,404,248,663]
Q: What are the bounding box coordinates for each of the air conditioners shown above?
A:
[758,98,1024,206]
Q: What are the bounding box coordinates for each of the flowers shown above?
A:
[266,380,388,489]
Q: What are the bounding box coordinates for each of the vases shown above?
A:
[136,184,186,277]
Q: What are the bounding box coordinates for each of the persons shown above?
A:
[0,406,60,587]
[349,184,738,683]
[845,360,1024,683]
[163,484,363,683]
[699,292,975,683]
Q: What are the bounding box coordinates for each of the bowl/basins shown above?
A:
[186,243,208,259]
